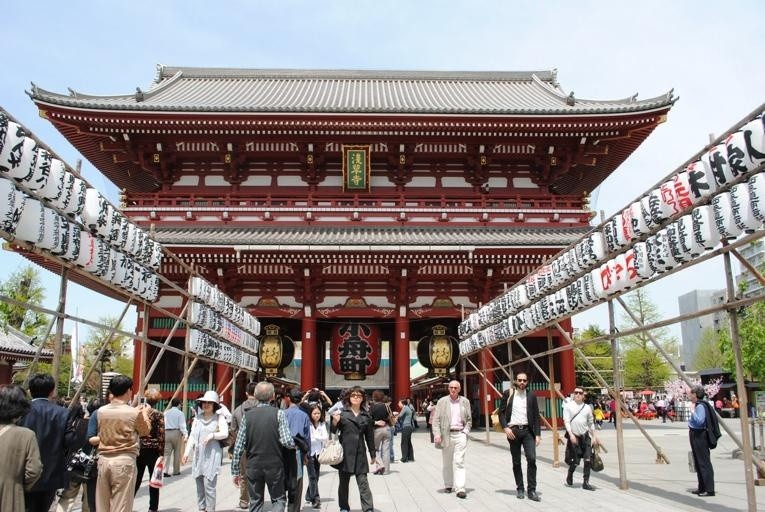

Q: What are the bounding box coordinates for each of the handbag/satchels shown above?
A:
[590,444,604,471]
[564,403,586,439]
[491,388,513,433]
[384,403,397,425]
[65,436,98,482]
[216,413,237,448]
[318,427,344,465]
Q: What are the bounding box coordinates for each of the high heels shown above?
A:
[374,467,385,474]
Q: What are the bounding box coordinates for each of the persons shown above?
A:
[609,394,616,428]
[56,401,85,512]
[724,397,731,408]
[0,383,43,512]
[59,394,88,410]
[273,392,289,410]
[432,380,473,499]
[283,389,311,512]
[24,372,78,512]
[217,402,232,465]
[88,385,115,512]
[331,386,376,512]
[95,375,152,511]
[597,395,611,419]
[387,396,392,403]
[499,372,541,502]
[716,400,723,416]
[181,390,229,512]
[427,399,438,443]
[593,404,605,430]
[626,395,682,423]
[407,397,415,416]
[329,389,350,416]
[563,387,596,491]
[192,394,202,417]
[396,400,413,463]
[305,404,328,509]
[134,388,165,512]
[424,398,430,428]
[83,396,99,512]
[231,381,298,512]
[731,394,739,417]
[688,386,721,497]
[227,382,258,509]
[369,390,392,475]
[163,398,188,478]
[383,395,395,463]
[300,388,332,422]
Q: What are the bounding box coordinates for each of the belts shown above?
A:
[514,425,528,430]
[450,429,463,432]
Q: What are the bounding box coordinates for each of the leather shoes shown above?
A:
[517,490,524,499]
[164,473,171,477]
[173,473,181,475]
[583,482,594,490]
[445,488,451,492]
[567,476,572,486]
[699,491,715,496]
[457,493,466,498]
[528,491,538,501]
[692,490,704,494]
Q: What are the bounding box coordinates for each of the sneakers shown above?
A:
[340,509,348,512]
[239,501,248,509]
[312,504,321,508]
[304,499,310,503]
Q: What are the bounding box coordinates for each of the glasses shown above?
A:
[450,387,457,389]
[574,391,583,395]
[518,379,527,382]
[351,394,363,398]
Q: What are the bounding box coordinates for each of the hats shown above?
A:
[196,391,222,410]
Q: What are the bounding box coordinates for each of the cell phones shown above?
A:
[139,396,146,409]
